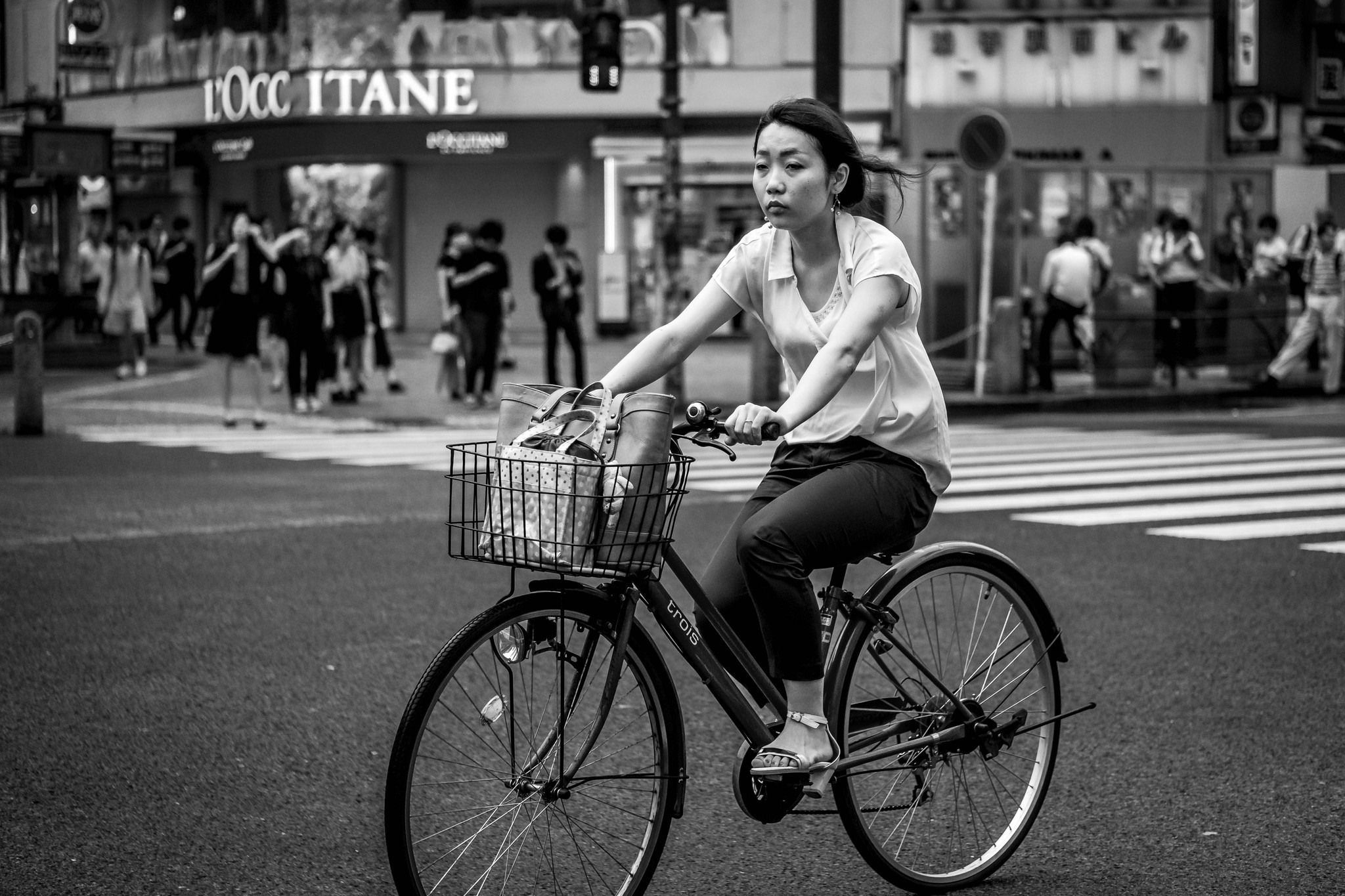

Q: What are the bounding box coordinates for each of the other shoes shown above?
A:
[1324,392,1340,400]
[388,383,402,393]
[1254,372,1280,386]
[450,390,461,401]
[1028,385,1051,393]
[270,383,283,392]
[479,392,499,409]
[500,361,518,371]
[251,418,266,430]
[353,383,364,394]
[291,397,323,414]
[464,393,480,410]
[224,417,236,428]
[136,360,148,378]
[329,390,357,405]
[116,366,135,380]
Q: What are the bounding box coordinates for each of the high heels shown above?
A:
[750,710,841,799]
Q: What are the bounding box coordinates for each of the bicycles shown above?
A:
[382,402,1096,896]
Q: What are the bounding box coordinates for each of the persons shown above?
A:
[570,98,954,776]
[81,200,587,430]
[1029,210,1345,400]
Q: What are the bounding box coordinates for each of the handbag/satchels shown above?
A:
[1099,263,1111,293]
[152,266,169,283]
[476,382,675,572]
[431,321,460,355]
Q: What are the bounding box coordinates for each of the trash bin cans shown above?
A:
[1088,273,1155,386]
[1232,274,1289,380]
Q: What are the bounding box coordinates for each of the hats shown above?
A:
[171,214,191,231]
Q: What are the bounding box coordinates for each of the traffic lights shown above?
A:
[579,11,624,93]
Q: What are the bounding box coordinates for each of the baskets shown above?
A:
[445,441,696,583]
